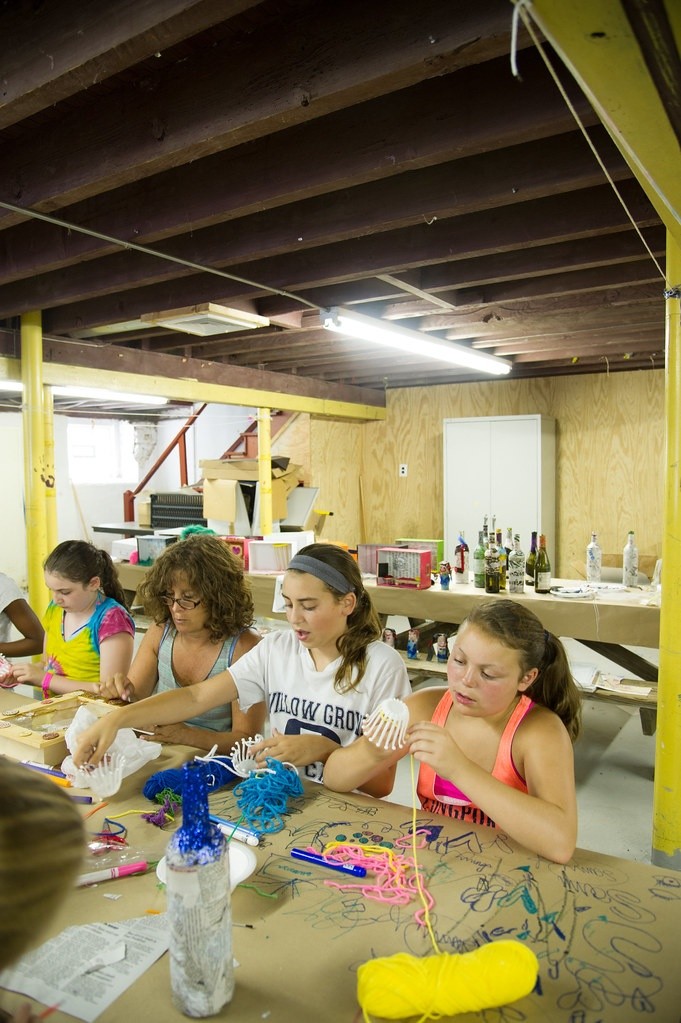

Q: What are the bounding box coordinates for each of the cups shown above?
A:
[81,752,125,797]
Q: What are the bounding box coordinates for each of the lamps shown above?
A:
[319,307,513,374]
[140,303,270,337]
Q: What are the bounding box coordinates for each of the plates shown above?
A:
[549,587,598,598]
[155,841,258,889]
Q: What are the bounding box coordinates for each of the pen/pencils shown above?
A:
[209,815,260,846]
[77,861,147,887]
[18,762,71,788]
[291,848,367,878]
[71,796,92,805]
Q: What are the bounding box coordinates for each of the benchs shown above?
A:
[133,613,659,710]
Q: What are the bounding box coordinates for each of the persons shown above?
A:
[0,752,84,970]
[323,599,581,863]
[0,571,45,658]
[9,540,134,700]
[97,533,266,755]
[71,543,412,799]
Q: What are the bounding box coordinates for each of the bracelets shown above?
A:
[42,673,53,699]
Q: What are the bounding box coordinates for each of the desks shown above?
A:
[1,686,681,1023]
[115,572,664,737]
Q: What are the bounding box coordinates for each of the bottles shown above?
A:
[622,531,639,588]
[164,761,237,1019]
[586,532,602,589]
[453,514,551,594]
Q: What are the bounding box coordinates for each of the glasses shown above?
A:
[158,591,206,610]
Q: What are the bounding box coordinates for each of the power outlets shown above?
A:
[398,464,408,476]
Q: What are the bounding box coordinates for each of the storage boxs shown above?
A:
[223,536,445,589]
[198,459,295,480]
